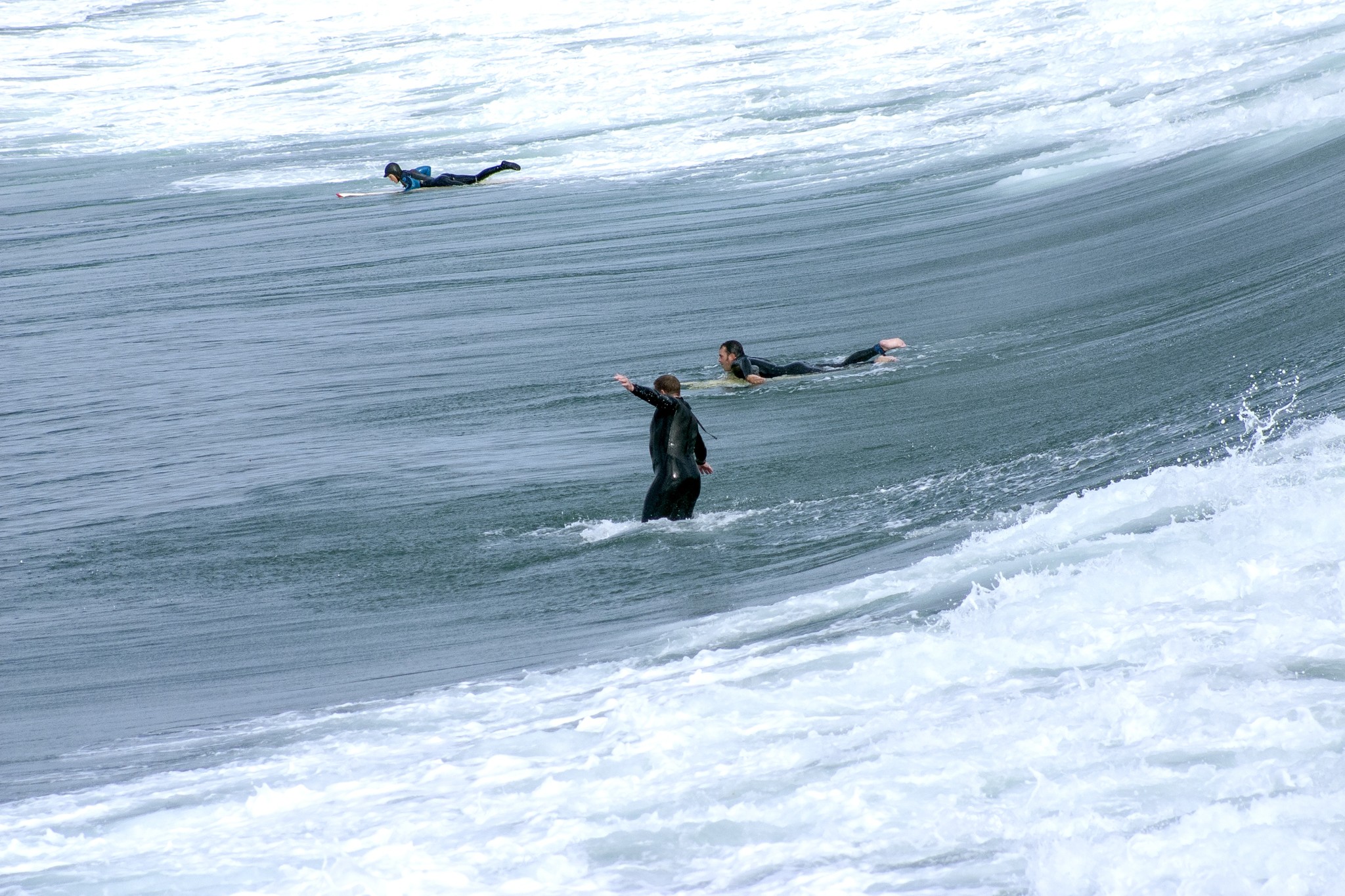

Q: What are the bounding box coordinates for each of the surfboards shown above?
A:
[679,373,815,387]
[335,174,533,198]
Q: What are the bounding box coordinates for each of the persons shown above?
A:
[611,372,714,521]
[717,338,906,385]
[383,160,522,193]
